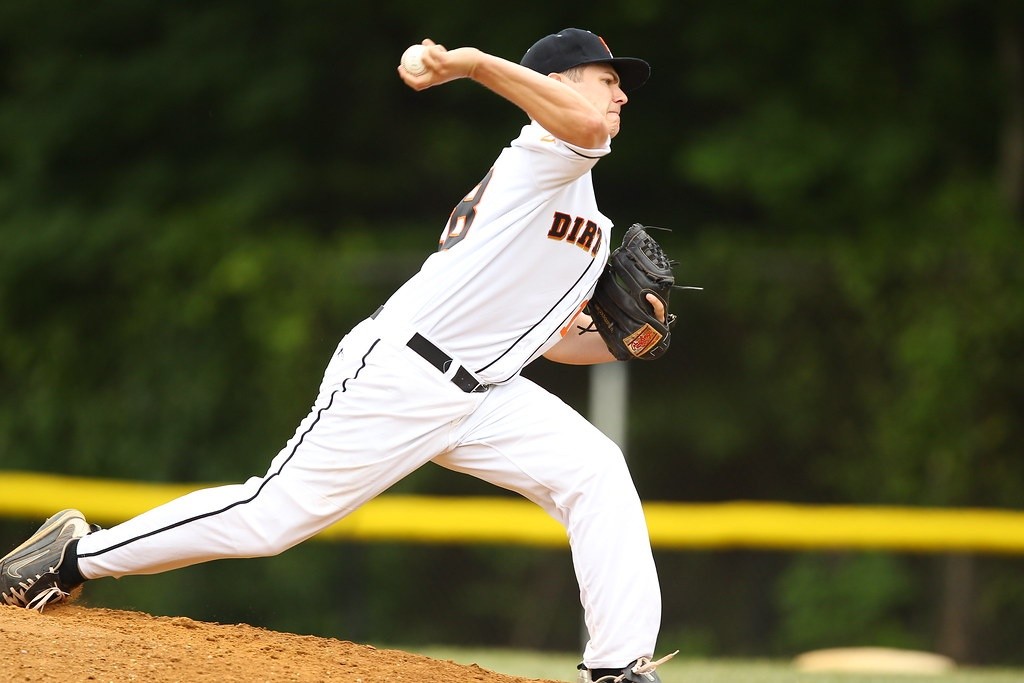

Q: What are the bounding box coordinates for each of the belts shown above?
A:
[370,304,490,393]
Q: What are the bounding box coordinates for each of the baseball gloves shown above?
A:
[584,221,678,363]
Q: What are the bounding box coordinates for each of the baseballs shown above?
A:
[400,44,431,77]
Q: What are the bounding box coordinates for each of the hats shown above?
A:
[520,28,651,93]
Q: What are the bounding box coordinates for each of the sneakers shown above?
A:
[0,509,100,615]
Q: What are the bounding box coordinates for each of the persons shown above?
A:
[0,27,681,683]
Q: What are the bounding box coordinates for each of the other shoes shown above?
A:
[578,650,680,683]
